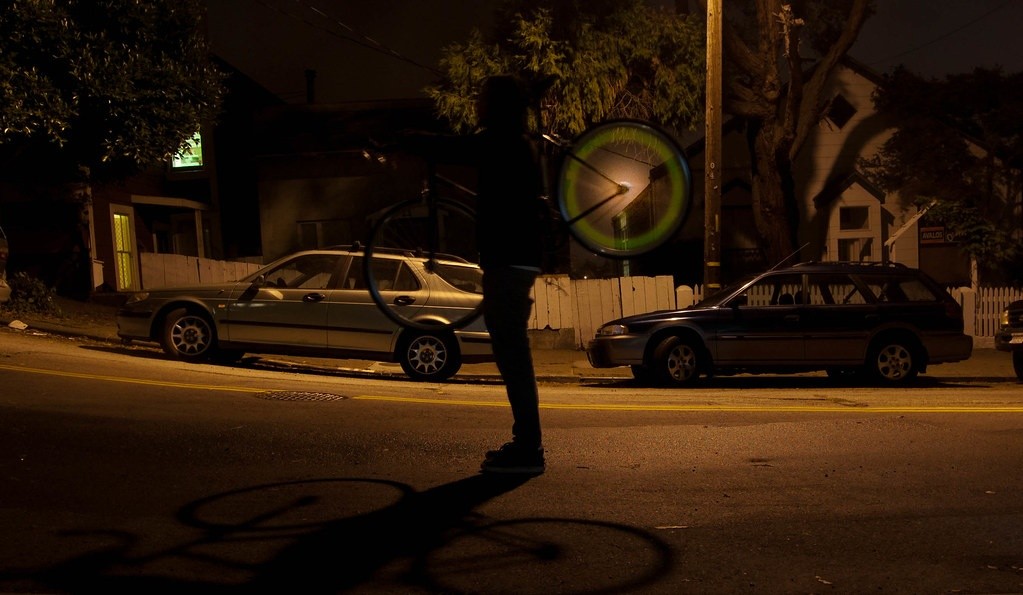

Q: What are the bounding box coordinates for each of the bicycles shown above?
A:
[360,78,691,332]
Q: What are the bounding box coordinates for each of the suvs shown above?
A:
[111,244,534,383]
[586,258,975,387]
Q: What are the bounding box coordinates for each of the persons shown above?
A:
[394,74,545,472]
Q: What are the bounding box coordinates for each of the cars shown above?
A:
[992,298,1023,385]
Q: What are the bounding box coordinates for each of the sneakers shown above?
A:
[480,435,545,474]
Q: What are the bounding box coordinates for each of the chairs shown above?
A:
[353,275,365,289]
[795,291,811,305]
[779,293,794,305]
